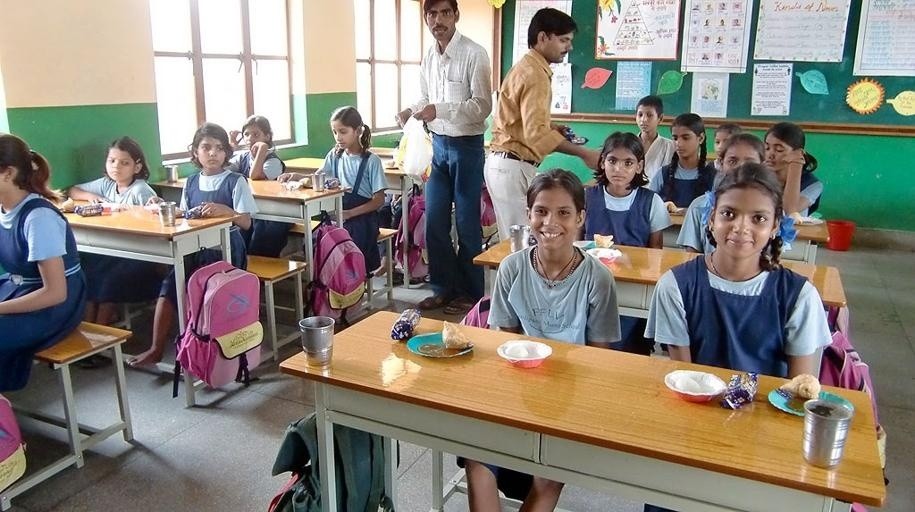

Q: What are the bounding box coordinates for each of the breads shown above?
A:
[663,200,677,213]
[778,371,822,402]
[789,210,803,227]
[591,232,615,249]
[441,318,467,351]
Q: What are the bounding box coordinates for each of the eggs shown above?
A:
[505,344,528,361]
[674,375,700,394]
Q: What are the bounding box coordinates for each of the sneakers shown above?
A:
[76,354,113,368]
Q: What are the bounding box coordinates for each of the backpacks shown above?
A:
[268,409,393,512]
[819,304,888,503]
[176,245,264,389]
[479,180,499,240]
[306,210,373,325]
[458,295,491,330]
[396,183,430,279]
[1,394,26,493]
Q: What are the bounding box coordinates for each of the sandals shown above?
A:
[418,293,478,315]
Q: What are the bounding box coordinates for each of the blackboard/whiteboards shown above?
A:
[490,0,915,138]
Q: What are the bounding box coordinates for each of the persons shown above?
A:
[67,135,161,368]
[582,95,825,257]
[124,121,261,370]
[483,4,602,243]
[643,163,835,512]
[0,132,88,392]
[277,105,386,272]
[455,166,622,512]
[227,114,286,260]
[393,0,494,316]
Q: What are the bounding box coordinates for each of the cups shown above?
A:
[159,200,177,227]
[164,165,179,183]
[509,224,531,252]
[299,316,335,366]
[311,173,326,192]
[802,399,854,468]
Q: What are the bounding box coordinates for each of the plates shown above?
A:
[583,241,595,248]
[407,332,474,359]
[768,388,854,417]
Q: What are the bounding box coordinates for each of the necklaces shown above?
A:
[710,253,723,280]
[532,243,579,290]
[536,247,575,287]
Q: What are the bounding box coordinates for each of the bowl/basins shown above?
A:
[664,369,727,402]
[496,340,553,368]
[585,248,620,264]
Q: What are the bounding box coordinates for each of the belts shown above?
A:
[492,150,541,169]
